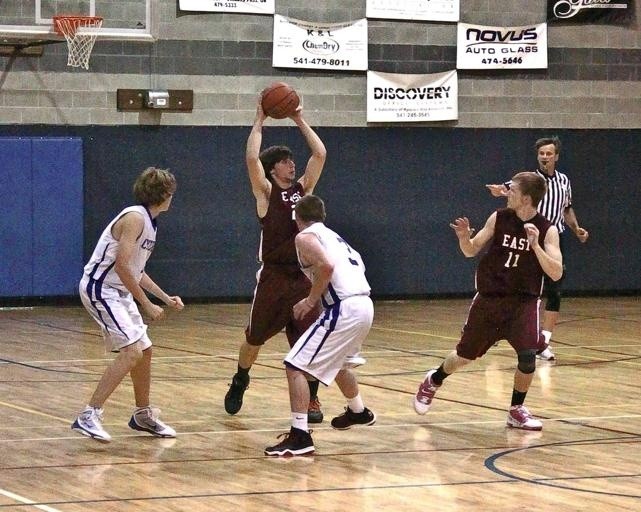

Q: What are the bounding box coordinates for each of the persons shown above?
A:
[264,197,378,462]
[223,91,330,424]
[70,167,186,442]
[459,136,589,359]
[413,172,564,432]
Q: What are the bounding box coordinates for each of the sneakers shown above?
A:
[333,409,377,428]
[69,405,113,445]
[415,368,437,413]
[127,405,178,440]
[265,430,316,456]
[506,407,542,429]
[309,381,323,422]
[223,369,251,415]
[534,332,555,361]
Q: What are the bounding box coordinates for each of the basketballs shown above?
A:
[261,81,299,120]
